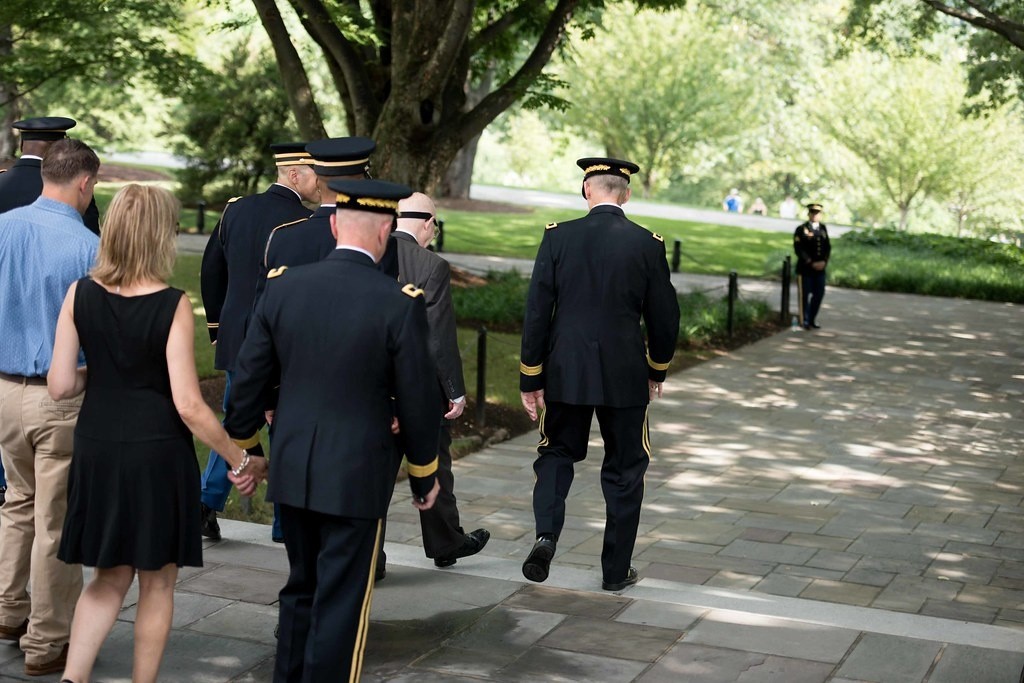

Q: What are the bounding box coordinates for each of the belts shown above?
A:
[0,372,47,386]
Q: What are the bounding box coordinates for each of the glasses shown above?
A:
[433,220,440,238]
[174,222,181,235]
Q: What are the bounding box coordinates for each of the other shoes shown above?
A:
[803,320,810,330]
[25,641,70,674]
[809,321,821,329]
[200,504,223,540]
[0,617,30,641]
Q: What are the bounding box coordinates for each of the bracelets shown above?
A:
[232,449,249,476]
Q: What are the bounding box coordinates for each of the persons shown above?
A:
[47,184,270,683]
[0,138,101,676]
[518,158,681,591]
[780,196,800,218]
[0,117,101,237]
[392,192,490,567]
[723,189,743,213]
[224,181,440,683]
[201,144,319,543]
[747,198,766,215]
[793,203,830,330]
[255,137,399,582]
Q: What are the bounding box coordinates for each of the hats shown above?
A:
[270,142,315,170]
[305,136,376,176]
[807,204,823,214]
[11,117,77,141]
[326,177,414,232]
[577,157,640,200]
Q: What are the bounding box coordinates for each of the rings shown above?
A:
[652,386,655,388]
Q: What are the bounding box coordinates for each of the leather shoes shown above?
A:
[434,529,490,567]
[603,568,638,591]
[523,534,557,583]
